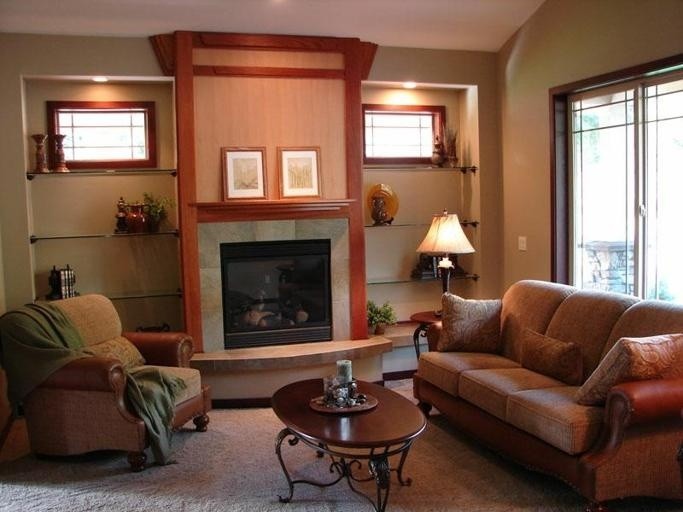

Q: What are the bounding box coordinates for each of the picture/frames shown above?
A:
[220,146,269,202]
[277,145,324,202]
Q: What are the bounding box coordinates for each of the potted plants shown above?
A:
[367,299,401,336]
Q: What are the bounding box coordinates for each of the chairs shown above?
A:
[1,293,213,472]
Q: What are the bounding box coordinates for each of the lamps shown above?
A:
[416,208,474,318]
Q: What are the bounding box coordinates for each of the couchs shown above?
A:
[413,280,683,512]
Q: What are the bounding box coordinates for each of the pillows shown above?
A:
[437,291,503,354]
[519,324,584,387]
[575,333,683,406]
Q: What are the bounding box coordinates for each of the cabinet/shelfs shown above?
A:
[28,168,183,304]
[362,165,480,287]
[191,336,393,401]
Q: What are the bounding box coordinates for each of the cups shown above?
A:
[336,359,353,383]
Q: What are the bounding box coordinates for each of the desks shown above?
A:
[410,311,441,362]
